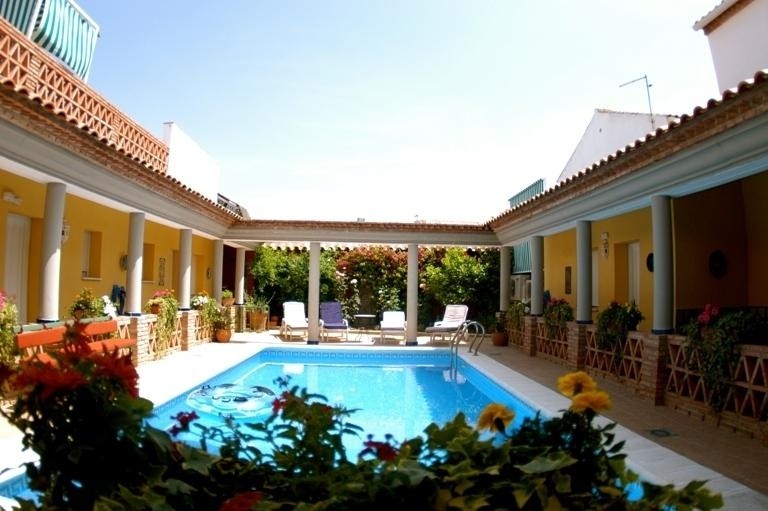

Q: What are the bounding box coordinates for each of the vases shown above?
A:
[548,311,562,319]
[194,304,203,309]
[607,320,636,330]
[700,328,715,340]
[73,309,83,318]
[151,304,160,313]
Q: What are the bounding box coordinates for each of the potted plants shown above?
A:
[492,323,508,346]
[223,290,235,306]
[208,314,234,342]
[245,291,275,330]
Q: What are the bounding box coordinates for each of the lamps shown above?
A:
[61,217,70,245]
[4,191,21,205]
[599,231,609,257]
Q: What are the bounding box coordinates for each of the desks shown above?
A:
[353,314,376,342]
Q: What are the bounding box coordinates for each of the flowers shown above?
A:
[606,301,640,318]
[76,287,95,304]
[547,298,567,306]
[193,291,208,306]
[154,289,174,298]
[696,304,720,325]
[0,292,17,325]
[0,323,724,511]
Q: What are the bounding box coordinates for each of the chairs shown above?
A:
[280,301,308,342]
[319,302,350,341]
[425,305,469,343]
[379,311,407,344]
